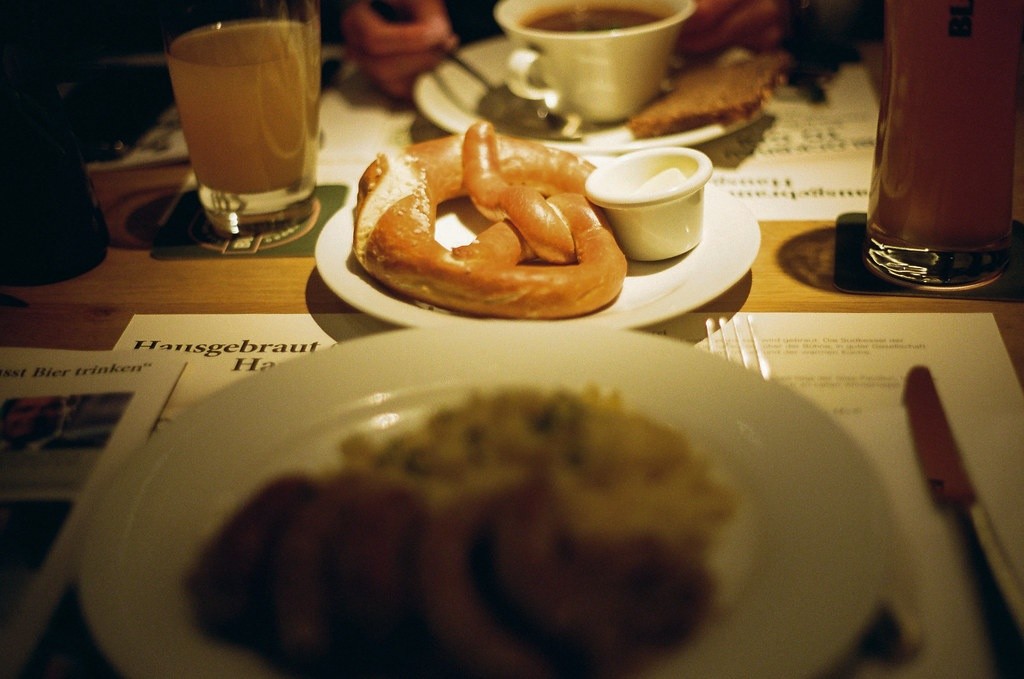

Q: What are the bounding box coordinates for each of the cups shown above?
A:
[165,18,319,237]
[864,0,1024,290]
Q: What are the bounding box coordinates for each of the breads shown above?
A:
[631,61,766,138]
[351,121,627,318]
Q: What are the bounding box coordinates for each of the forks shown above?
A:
[706,311,771,383]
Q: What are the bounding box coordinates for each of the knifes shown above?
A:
[903,365,1024,668]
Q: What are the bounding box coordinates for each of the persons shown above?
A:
[339,0,865,104]
[0,395,81,450]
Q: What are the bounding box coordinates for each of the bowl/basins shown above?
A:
[585,146,714,262]
[494,0,697,124]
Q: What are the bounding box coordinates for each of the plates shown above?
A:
[75,322,895,679]
[412,36,763,155]
[314,157,761,327]
